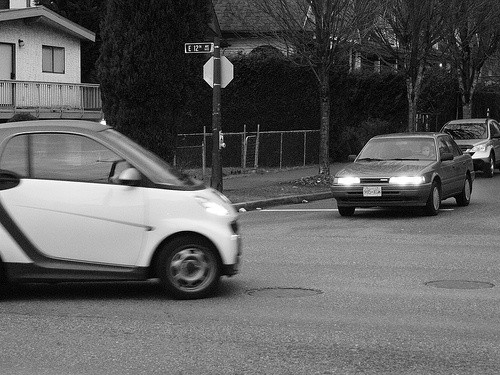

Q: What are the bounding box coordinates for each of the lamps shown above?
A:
[18,39,25,47]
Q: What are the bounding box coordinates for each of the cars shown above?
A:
[0,119,242,300]
[438,118,500,178]
[330,131,477,216]
